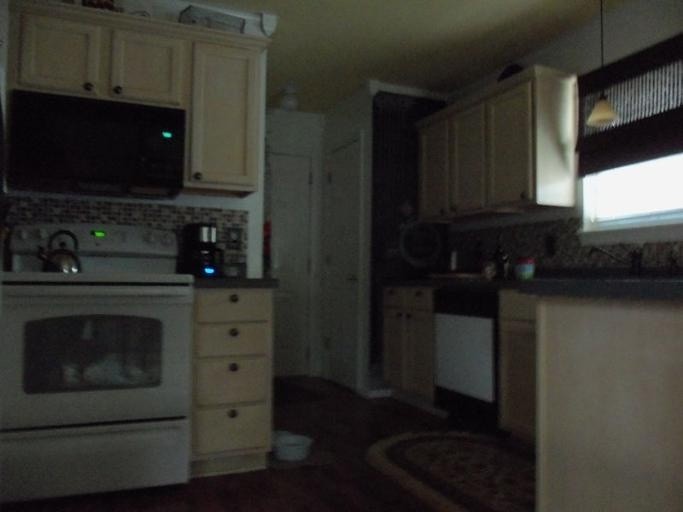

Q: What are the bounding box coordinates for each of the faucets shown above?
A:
[589,243,643,273]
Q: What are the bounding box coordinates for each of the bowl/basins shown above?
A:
[221,262,241,279]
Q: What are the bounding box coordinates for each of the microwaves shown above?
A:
[4,87,186,202]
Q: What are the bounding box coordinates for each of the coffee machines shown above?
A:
[178,220,224,276]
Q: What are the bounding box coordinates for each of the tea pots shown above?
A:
[35,227,84,275]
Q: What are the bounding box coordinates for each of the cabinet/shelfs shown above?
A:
[412,90,486,217]
[12,3,189,104]
[497,288,536,445]
[379,287,437,398]
[183,34,263,189]
[487,63,582,210]
[190,279,277,476]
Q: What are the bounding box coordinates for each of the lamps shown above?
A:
[586,1,621,128]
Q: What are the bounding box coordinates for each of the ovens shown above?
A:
[0,286,196,505]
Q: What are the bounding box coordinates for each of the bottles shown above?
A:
[513,257,536,282]
[446,248,458,272]
